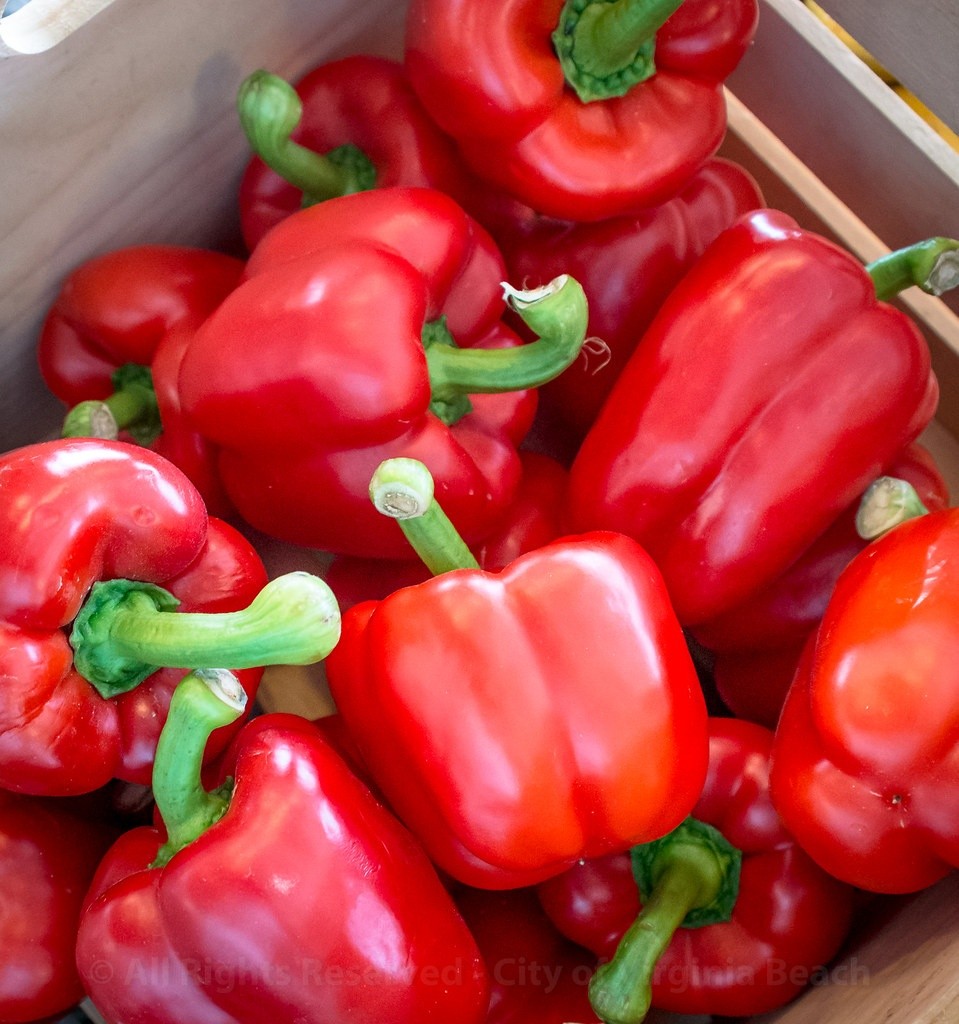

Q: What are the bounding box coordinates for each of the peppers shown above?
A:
[0,0,959,1024]
